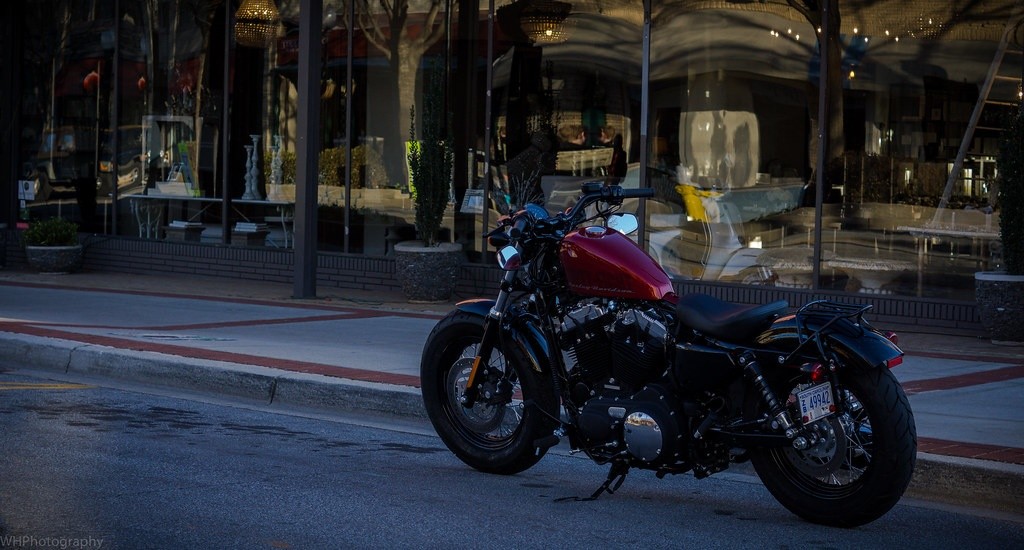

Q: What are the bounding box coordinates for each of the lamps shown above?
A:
[233,0,279,45]
[522,15,579,44]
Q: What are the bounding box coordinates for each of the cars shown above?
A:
[811,151,902,190]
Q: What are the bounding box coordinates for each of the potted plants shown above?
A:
[394,55,462,304]
[975,77,1024,348]
[23,218,86,275]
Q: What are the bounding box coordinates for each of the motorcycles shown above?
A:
[420,182,916,527]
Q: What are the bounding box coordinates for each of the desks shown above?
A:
[895,226,1001,297]
[122,193,296,251]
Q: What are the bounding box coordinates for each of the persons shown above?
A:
[557,125,628,185]
